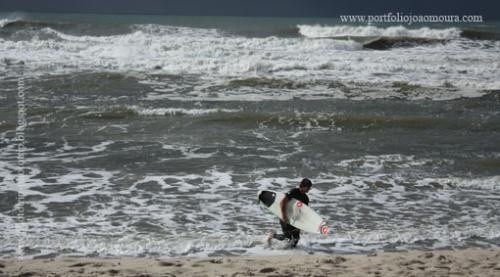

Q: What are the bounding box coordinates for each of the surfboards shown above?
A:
[256,188,331,237]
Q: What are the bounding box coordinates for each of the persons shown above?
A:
[266,178,313,249]
